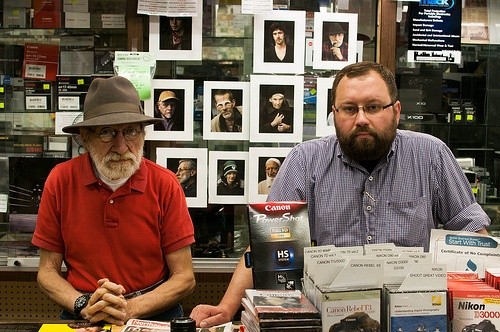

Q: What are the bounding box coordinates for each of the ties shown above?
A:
[173,32,182,50]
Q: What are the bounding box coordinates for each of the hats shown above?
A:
[267,85,285,100]
[223,161,239,177]
[325,23,347,35]
[159,91,180,101]
[62,76,164,134]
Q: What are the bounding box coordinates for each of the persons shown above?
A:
[175,159,197,197]
[266,22,294,63]
[31,76,195,326]
[165,17,191,50]
[258,158,281,194]
[322,23,347,61]
[259,87,293,133]
[211,89,242,132]
[217,161,244,195]
[189,62,491,328]
[154,91,184,131]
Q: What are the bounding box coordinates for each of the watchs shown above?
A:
[73,294,91,321]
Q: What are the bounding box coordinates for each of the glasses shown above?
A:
[85,125,141,142]
[334,103,393,119]
[177,168,194,173]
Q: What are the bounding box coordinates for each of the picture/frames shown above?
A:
[143,0,364,209]
